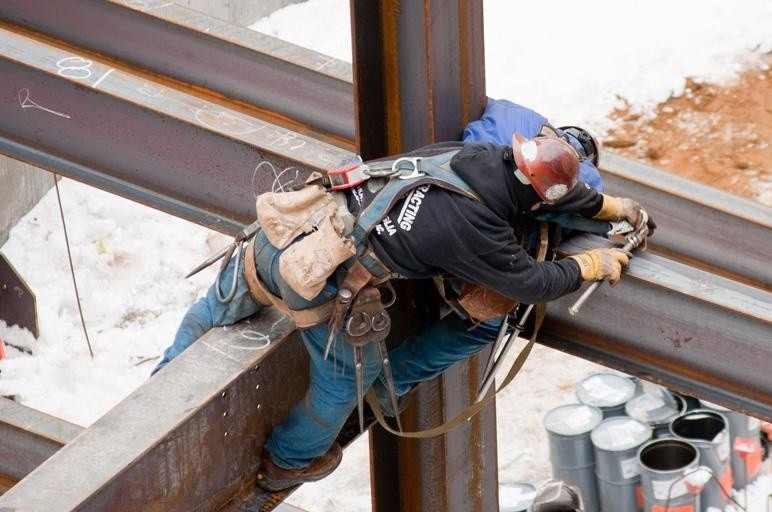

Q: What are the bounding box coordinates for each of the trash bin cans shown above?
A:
[531,482,583,512]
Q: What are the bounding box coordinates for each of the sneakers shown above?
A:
[255,443,344,491]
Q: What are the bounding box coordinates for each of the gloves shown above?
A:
[567,248,634,288]
[595,192,643,222]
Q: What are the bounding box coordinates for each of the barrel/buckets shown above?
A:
[496,370,763,512]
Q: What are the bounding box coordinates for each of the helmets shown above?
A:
[511,132,580,205]
[557,121,602,167]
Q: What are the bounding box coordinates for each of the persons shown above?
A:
[326,97,603,449]
[147,133,658,492]
